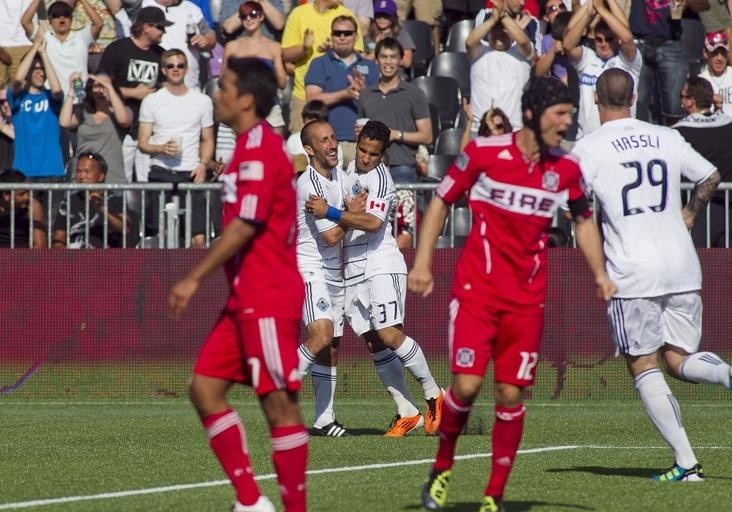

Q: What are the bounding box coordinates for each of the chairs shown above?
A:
[0,0,729,251]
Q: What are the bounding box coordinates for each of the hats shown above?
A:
[373,0,397,17]
[521,78,576,149]
[139,6,175,26]
[703,33,729,53]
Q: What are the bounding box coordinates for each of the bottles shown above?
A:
[73,78,88,101]
[185,13,197,49]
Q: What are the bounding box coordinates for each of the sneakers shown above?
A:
[647,461,706,481]
[383,413,424,437]
[421,468,455,511]
[230,497,279,512]
[310,419,360,437]
[424,386,447,434]
[479,494,506,512]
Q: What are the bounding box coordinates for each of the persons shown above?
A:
[167,56,310,512]
[0,0,732,249]
[297,119,368,437]
[305,120,445,437]
[407,78,618,512]
[562,68,732,482]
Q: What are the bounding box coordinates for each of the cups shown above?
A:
[170,135,184,154]
[356,118,371,130]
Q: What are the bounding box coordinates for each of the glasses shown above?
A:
[543,3,566,14]
[163,63,187,69]
[680,92,691,99]
[594,36,618,43]
[238,10,265,20]
[48,12,72,19]
[331,30,358,37]
